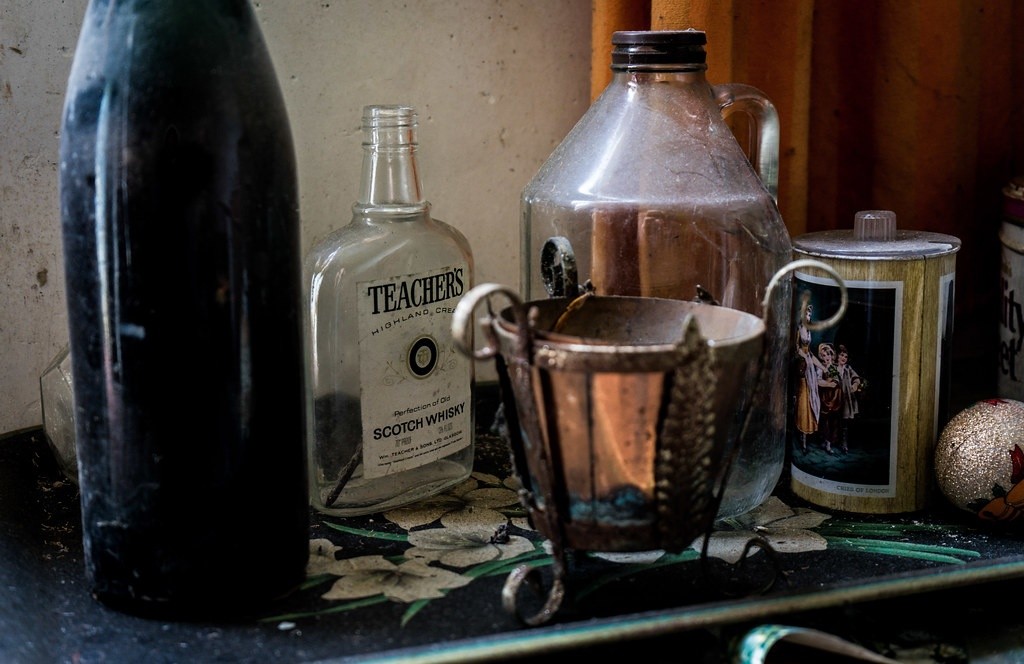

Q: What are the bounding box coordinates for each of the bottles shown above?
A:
[794,208,961,514]
[521,28,792,520]
[303,104,476,516]
[59,0,313,627]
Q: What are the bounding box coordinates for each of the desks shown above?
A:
[0,399,1024,664]
